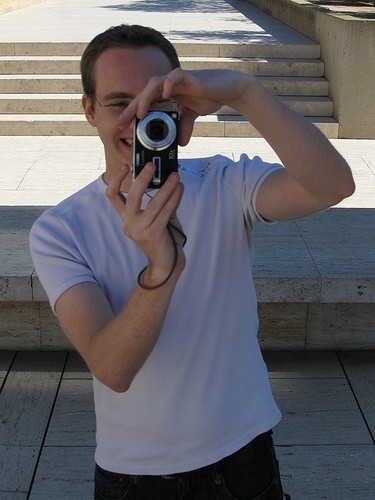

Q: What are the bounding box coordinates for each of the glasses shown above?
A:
[86,91,177,113]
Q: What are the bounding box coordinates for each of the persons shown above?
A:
[29,24,356,500]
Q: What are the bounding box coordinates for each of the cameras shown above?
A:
[132,110,181,191]
